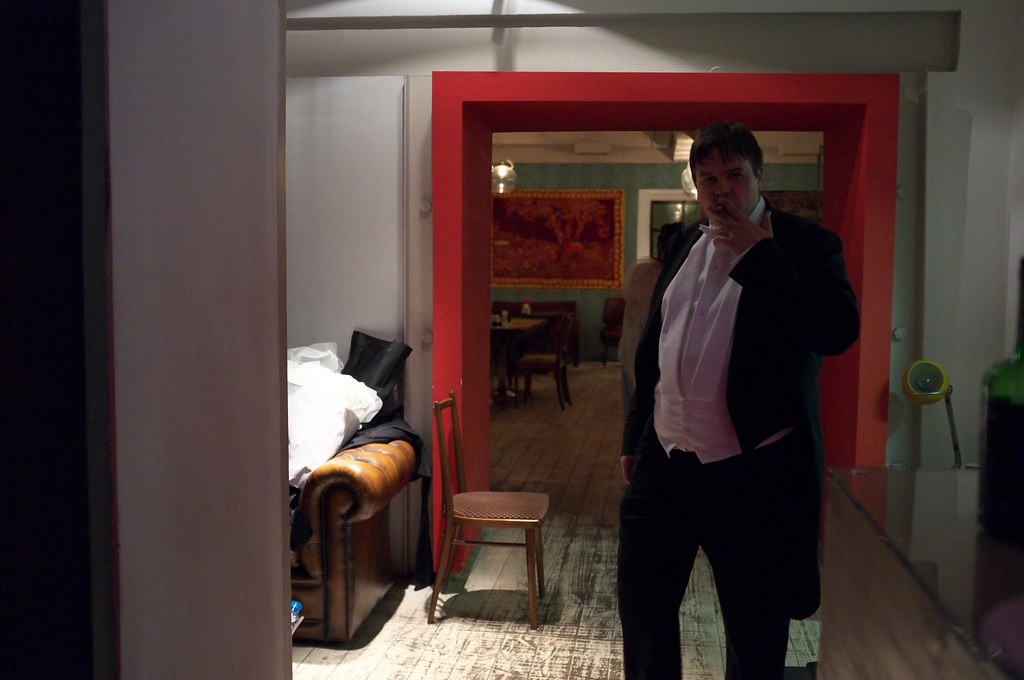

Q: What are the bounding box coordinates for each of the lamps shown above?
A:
[491,159,518,193]
[904,360,964,470]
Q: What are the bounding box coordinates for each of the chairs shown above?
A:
[600,297,626,368]
[428,392,549,631]
[513,317,581,409]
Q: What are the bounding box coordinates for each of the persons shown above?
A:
[617,221,685,417]
[616,124,862,680]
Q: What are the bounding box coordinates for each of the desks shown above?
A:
[497,317,544,402]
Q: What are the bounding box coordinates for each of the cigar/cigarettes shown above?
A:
[716,206,722,211]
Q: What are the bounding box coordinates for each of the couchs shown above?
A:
[288,330,424,646]
[493,299,580,367]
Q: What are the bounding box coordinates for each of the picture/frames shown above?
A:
[490,187,625,290]
